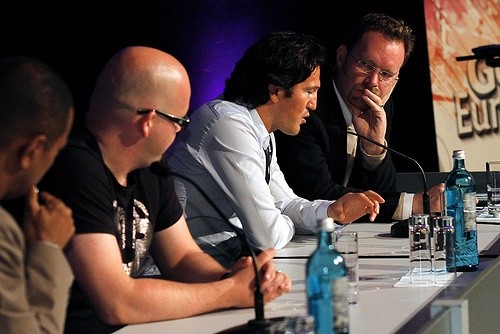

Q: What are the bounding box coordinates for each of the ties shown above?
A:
[264,135,273,185]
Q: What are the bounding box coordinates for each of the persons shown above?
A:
[141,31,385,276]
[274,13,446,224]
[0,45,291,334]
[0,59,77,334]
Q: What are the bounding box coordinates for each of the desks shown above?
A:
[111,221,500,334]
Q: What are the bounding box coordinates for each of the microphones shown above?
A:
[328,126,442,239]
[148,161,289,334]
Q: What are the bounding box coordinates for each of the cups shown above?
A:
[487,160,500,205]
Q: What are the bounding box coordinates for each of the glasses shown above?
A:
[350,51,398,83]
[137,109,190,136]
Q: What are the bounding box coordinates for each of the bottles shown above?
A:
[430,215,459,282]
[407,214,432,276]
[444,150,480,274]
[334,232,359,304]
[305,217,350,334]
